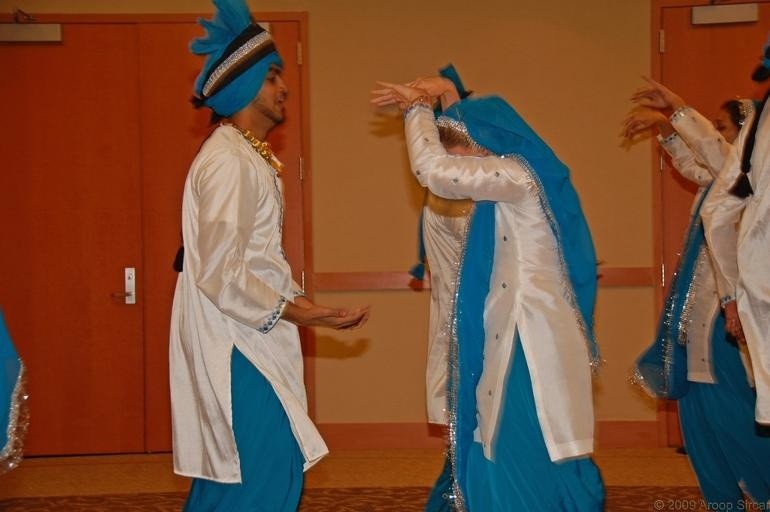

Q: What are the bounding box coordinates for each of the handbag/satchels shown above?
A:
[634,337,691,401]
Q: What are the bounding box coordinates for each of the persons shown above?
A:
[698,39,769,426]
[368,62,609,511]
[165,0,373,511]
[620,74,769,511]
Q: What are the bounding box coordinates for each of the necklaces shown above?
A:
[221,120,285,174]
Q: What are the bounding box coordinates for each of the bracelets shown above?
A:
[409,94,433,106]
